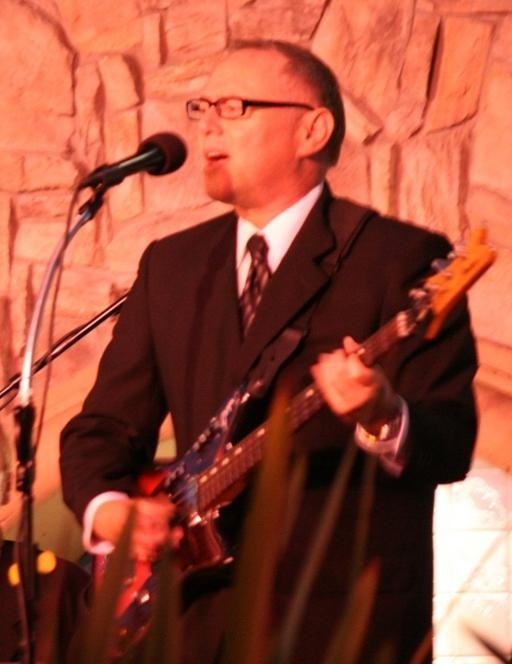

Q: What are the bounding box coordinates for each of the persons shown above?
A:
[59,39,480,664]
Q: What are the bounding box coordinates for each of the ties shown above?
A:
[239,234,271,343]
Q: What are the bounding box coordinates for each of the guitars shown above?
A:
[93,221,498,661]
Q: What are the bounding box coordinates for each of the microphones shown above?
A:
[78,132,187,190]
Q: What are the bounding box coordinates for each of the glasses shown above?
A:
[186,97,314,120]
